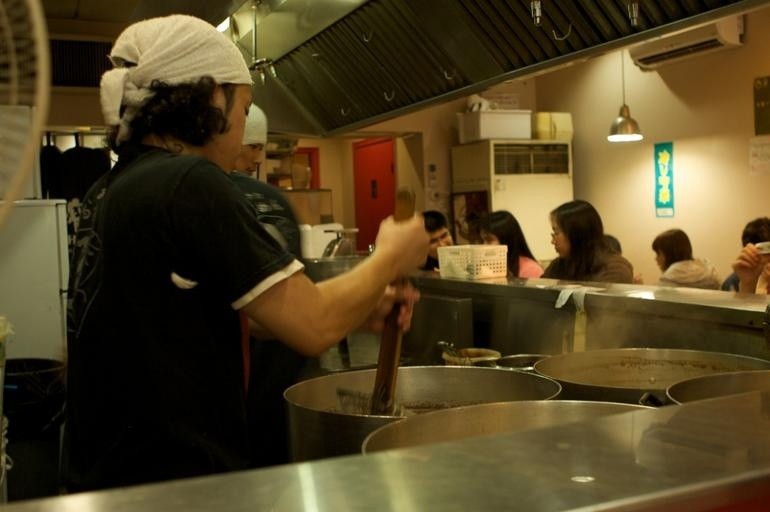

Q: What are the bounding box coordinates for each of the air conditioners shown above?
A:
[626,14,744,71]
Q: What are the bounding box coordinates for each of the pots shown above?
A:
[285,348,769,456]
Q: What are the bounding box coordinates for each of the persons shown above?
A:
[722,215,770,296]
[600,234,645,284]
[540,200,635,286]
[231,100,304,468]
[417,209,454,273]
[60,12,431,496]
[651,229,722,291]
[476,211,544,279]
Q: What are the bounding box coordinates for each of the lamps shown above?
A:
[606,45,644,143]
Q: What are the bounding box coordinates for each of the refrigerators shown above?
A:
[0,199,70,369]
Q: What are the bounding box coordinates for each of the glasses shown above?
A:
[550,231,562,237]
[429,232,449,243]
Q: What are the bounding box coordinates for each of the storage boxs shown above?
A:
[457,107,532,145]
